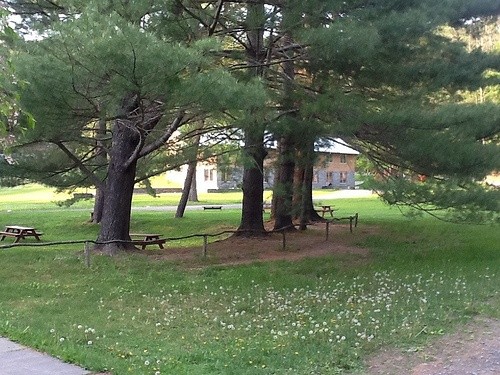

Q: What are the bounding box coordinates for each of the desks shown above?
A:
[1,226,40,243]
[128,234,164,250]
[314,205,335,218]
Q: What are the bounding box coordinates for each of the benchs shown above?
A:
[24,231,42,236]
[0,232,21,238]
